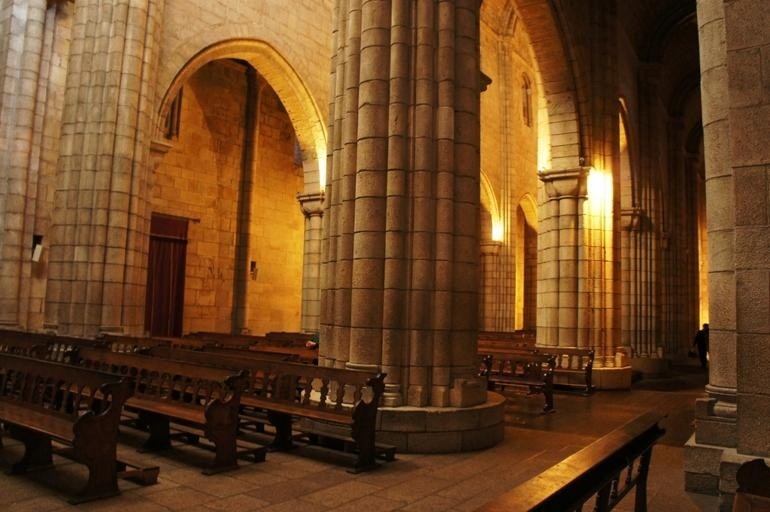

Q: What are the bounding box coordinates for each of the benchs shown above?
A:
[0,328,594,505]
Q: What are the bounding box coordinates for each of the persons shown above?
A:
[689,323,709,372]
